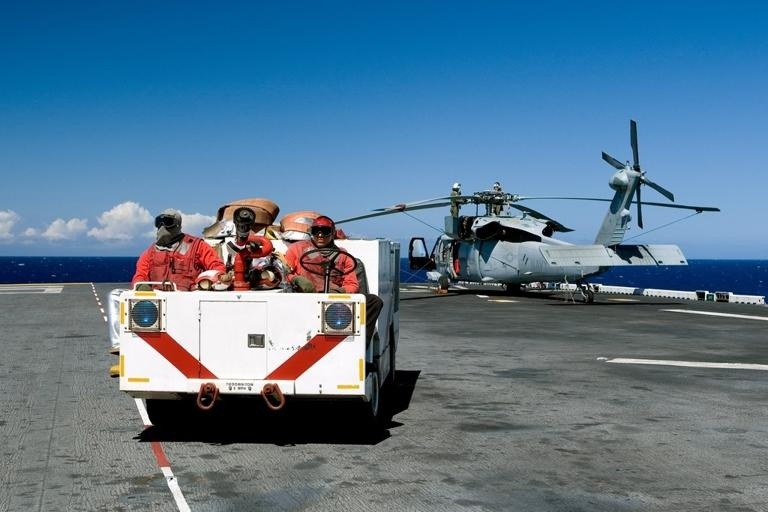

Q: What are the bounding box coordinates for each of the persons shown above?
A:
[107,207,229,378]
[283,215,384,353]
[448,182,463,218]
[491,181,505,216]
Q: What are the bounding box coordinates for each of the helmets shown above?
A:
[310,217,332,227]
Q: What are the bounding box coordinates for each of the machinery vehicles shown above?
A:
[104,233,406,426]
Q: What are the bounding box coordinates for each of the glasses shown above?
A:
[155,215,176,228]
[310,226,332,235]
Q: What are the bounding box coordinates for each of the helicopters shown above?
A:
[331,118,725,303]
